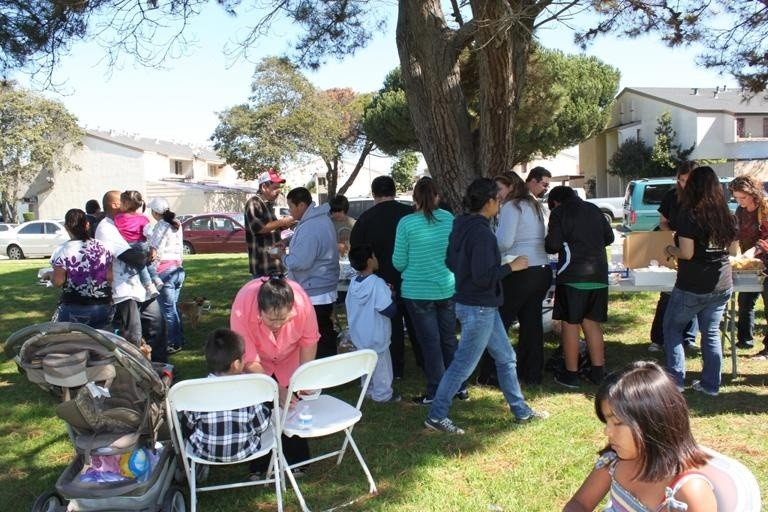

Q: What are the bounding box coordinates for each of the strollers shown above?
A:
[0,323,178,473]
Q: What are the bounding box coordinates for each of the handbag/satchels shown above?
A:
[622,230,678,269]
[545,336,591,376]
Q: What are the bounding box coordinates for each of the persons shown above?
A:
[648,159,768,394]
[178,328,273,485]
[561,359,717,512]
[245,171,357,360]
[346,164,614,435]
[228,270,322,486]
[43,190,185,361]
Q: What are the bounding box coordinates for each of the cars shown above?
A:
[0,223,18,231]
[176,215,194,222]
[348,197,414,220]
[272,206,291,220]
[181,213,293,254]
[0,219,71,260]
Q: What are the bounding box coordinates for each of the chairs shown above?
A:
[166,348,379,510]
[687,441,762,512]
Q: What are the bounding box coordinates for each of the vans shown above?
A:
[623,177,738,232]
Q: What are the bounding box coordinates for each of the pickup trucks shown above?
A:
[543,186,625,223]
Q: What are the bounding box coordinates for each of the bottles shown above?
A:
[297,404,312,430]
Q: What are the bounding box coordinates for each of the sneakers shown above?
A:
[424,417,466,435]
[292,467,307,478]
[644,331,768,396]
[156,278,163,291]
[166,342,182,355]
[364,359,610,406]
[246,471,263,481]
[148,284,160,299]
[515,411,549,423]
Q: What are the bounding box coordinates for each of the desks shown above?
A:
[604,264,763,380]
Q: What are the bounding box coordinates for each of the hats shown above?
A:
[259,169,286,186]
[146,196,170,214]
